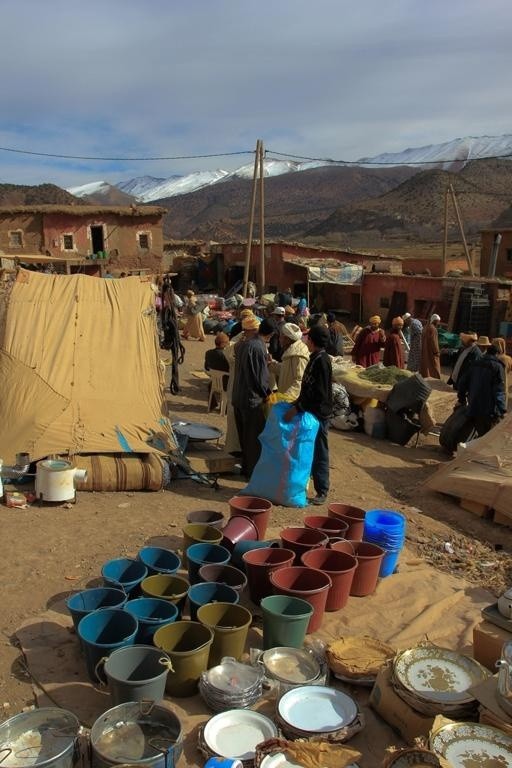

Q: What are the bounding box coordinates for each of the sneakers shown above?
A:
[314,495,327,504]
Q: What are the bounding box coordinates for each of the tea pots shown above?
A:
[494,639,511,719]
[496,586,512,619]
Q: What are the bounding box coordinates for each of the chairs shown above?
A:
[205,369,229,417]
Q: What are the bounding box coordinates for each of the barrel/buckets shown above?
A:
[364,407,385,436]
[66,495,406,705]
[215,298,225,311]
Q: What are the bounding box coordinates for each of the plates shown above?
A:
[429,722,512,768]
[198,634,489,768]
[170,422,223,441]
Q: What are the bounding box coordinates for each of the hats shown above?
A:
[272,307,286,315]
[477,336,493,346]
[282,323,302,341]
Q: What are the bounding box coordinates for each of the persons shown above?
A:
[283,323,334,506]
[446,330,511,437]
[232,319,274,479]
[154,278,344,409]
[352,312,441,380]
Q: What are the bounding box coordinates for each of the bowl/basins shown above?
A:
[1,696,186,767]
